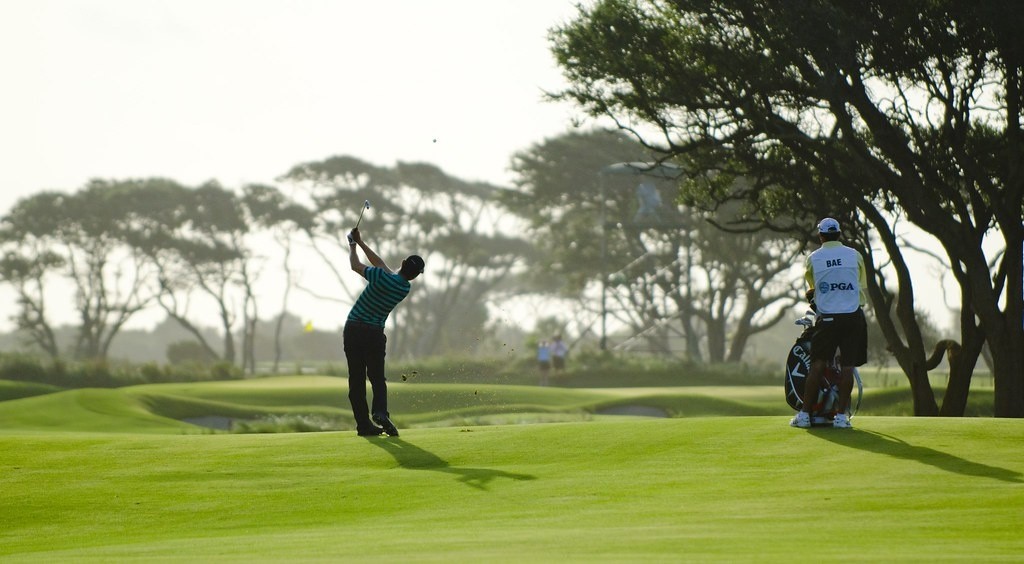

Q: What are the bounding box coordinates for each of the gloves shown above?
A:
[347,234,357,245]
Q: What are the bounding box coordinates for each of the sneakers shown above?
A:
[834,417,852,428]
[790,414,811,428]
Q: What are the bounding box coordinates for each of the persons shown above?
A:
[538,340,550,386]
[343,227,425,436]
[790,217,868,428]
[551,335,569,375]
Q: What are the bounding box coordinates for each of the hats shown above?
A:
[816,218,840,235]
[410,255,425,274]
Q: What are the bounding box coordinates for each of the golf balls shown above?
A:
[432,137,437,142]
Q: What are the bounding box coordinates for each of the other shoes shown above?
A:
[358,423,383,435]
[372,412,398,437]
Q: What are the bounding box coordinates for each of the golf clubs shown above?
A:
[356,200,369,228]
[794,311,816,330]
